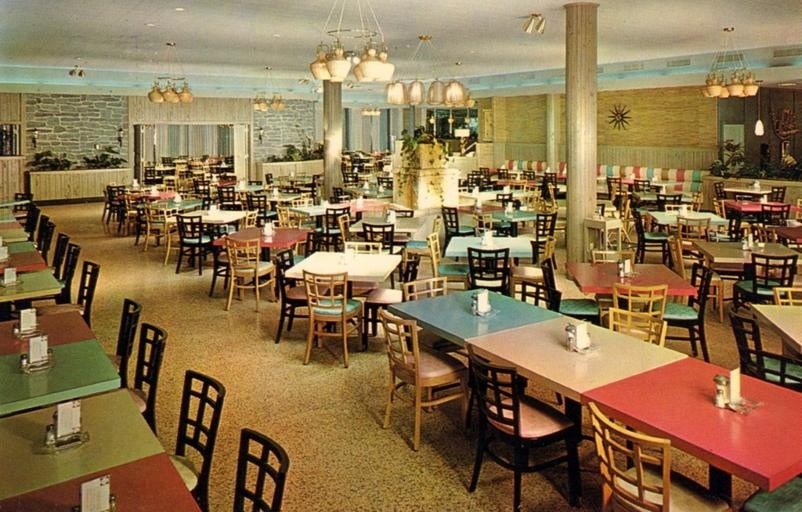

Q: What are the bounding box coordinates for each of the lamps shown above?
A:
[754,81,764,136]
[387,36,464,104]
[254,68,285,111]
[359,105,381,116]
[69,64,84,77]
[703,28,758,98]
[259,128,264,144]
[522,13,545,34]
[149,42,192,103]
[431,87,475,108]
[117,126,124,146]
[32,127,38,148]
[310,0,395,82]
